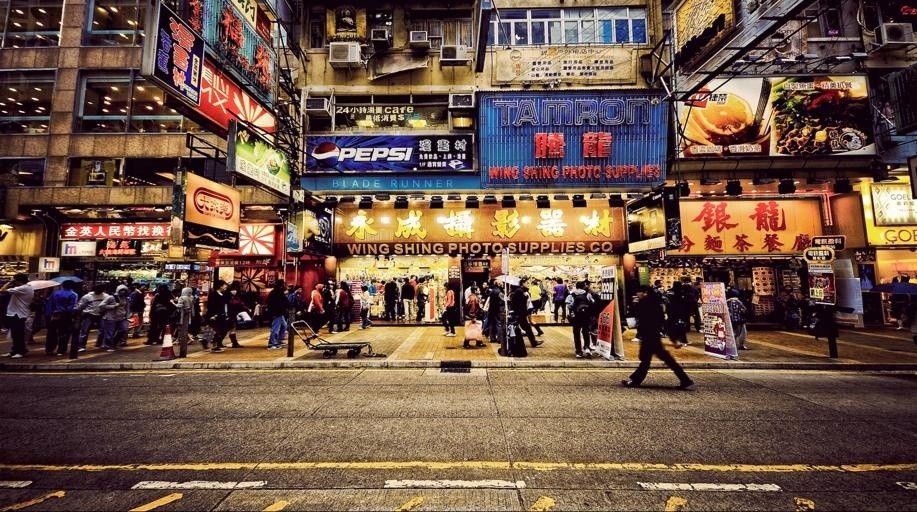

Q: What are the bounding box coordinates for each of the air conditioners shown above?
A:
[323,38,364,70]
[438,45,469,64]
[871,20,915,52]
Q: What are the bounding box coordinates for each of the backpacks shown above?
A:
[572,291,591,321]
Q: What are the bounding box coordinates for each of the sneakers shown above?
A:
[1,353,11,357]
[446,333,456,337]
[210,347,224,352]
[11,354,23,358]
[586,348,592,356]
[442,332,450,335]
[532,341,543,347]
[267,345,282,350]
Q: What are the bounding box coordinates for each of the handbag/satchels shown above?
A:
[360,307,369,318]
[464,320,482,341]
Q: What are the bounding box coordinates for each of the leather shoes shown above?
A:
[622,379,640,387]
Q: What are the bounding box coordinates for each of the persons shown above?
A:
[619,284,695,390]
[616,270,916,351]
[0,272,603,360]
[880,102,897,135]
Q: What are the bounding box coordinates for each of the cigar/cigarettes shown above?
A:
[632,295,637,298]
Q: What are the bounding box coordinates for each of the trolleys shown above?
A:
[289,319,370,359]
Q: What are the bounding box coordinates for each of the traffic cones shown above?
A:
[159,324,176,359]
